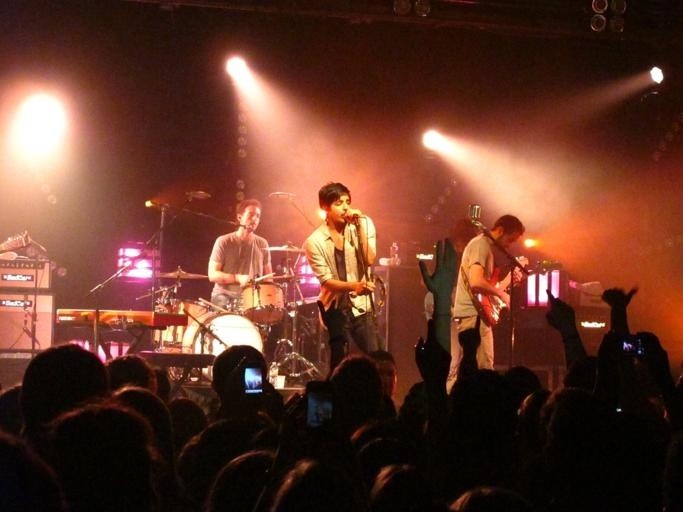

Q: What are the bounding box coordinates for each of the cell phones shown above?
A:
[303,395,333,427]
[241,366,265,393]
[621,338,642,355]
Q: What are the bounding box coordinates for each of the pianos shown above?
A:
[55,307,188,330]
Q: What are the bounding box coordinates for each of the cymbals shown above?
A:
[158,273,208,280]
[264,246,308,253]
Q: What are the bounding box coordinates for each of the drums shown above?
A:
[242,280,285,326]
[154,299,207,345]
[473,257,527,329]
[295,296,326,337]
[180,313,262,385]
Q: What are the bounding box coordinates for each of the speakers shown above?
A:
[0,291,56,353]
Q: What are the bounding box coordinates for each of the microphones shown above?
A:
[266,192,298,201]
[50,258,69,278]
[184,188,212,201]
[465,201,483,222]
[247,221,256,236]
[22,327,40,344]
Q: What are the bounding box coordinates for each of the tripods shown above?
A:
[265,299,328,386]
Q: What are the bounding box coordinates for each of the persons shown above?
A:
[303,183,381,368]
[2,238,682,512]
[446,215,527,394]
[209,197,274,313]
